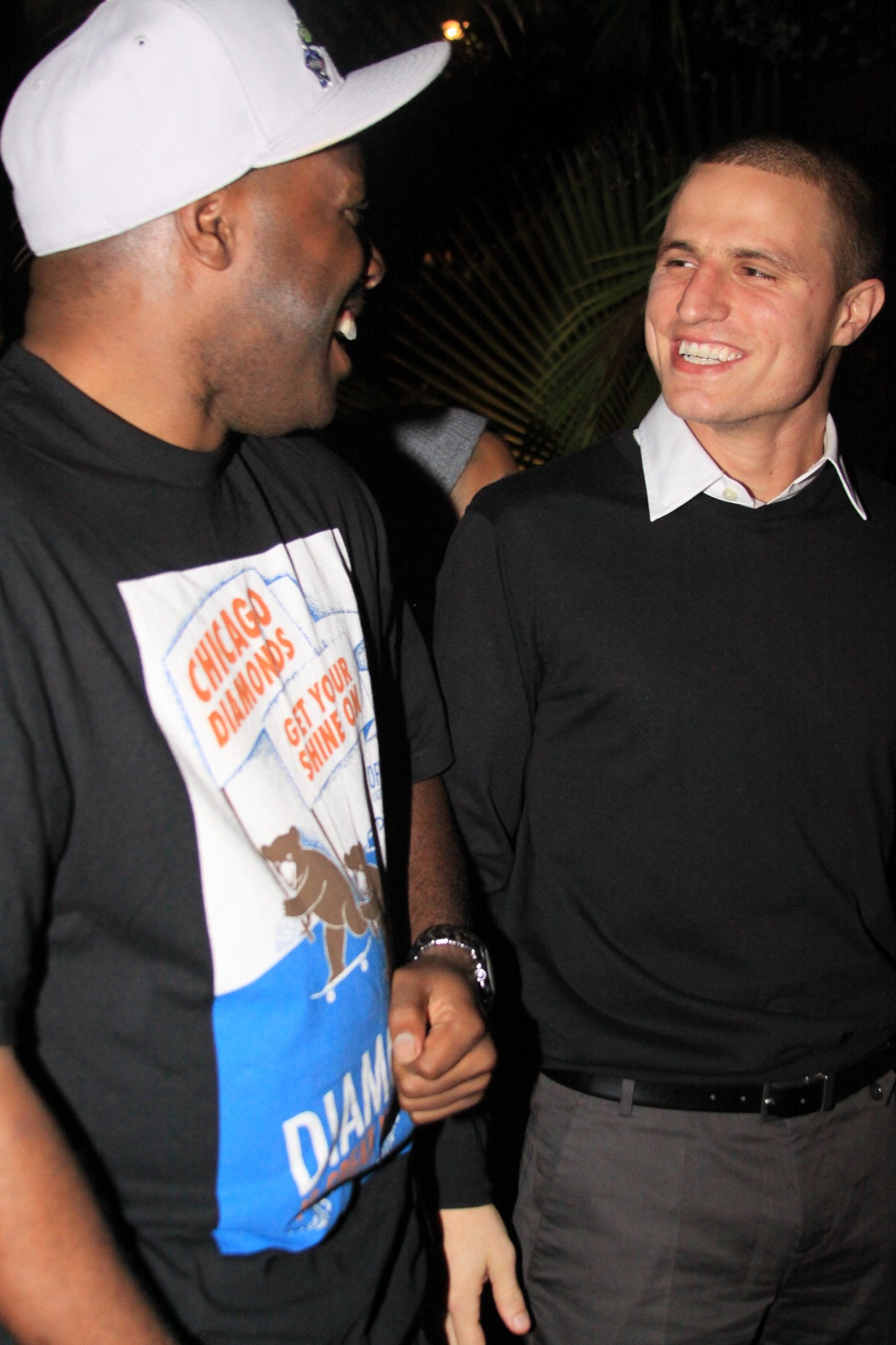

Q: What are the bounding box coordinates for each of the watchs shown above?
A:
[410,924,497,996]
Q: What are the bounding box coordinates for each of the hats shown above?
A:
[0,0,451,256]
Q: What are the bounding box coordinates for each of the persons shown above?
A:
[429,134,896,1345]
[0,0,500,1345]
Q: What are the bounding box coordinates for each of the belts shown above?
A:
[540,1047,895,1123]
